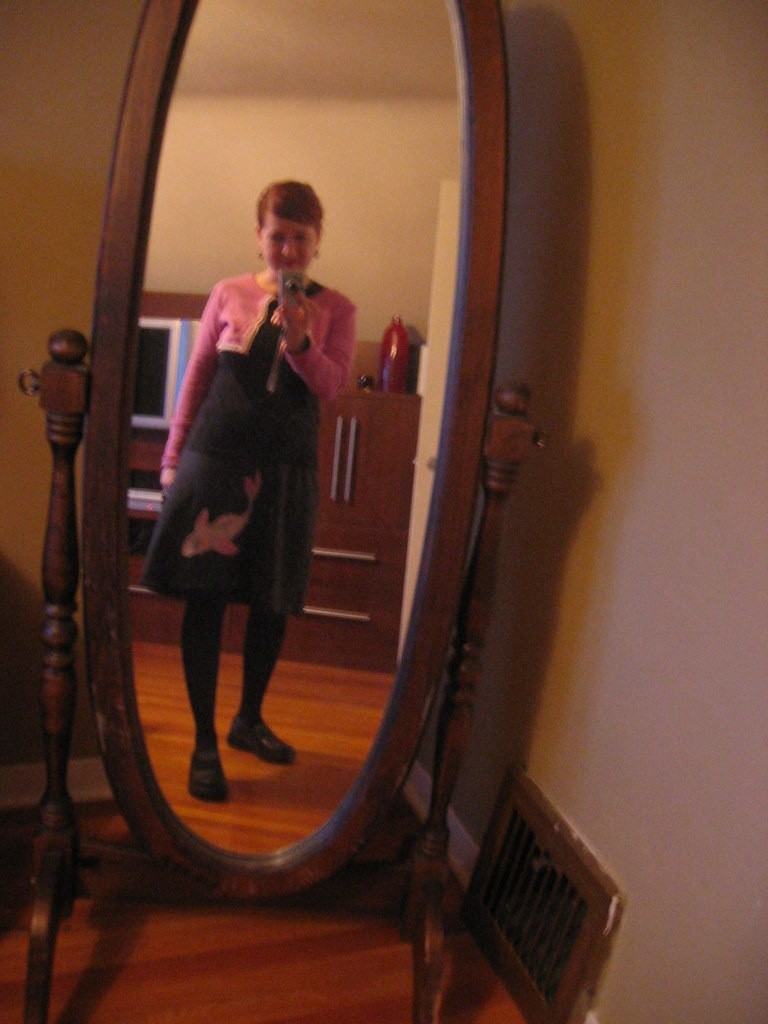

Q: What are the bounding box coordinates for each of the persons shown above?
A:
[146,177,365,797]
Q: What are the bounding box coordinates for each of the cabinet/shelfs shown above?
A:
[128,292,422,671]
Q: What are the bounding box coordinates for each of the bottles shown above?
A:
[378,316,410,394]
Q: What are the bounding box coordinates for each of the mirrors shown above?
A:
[11,1,537,1024]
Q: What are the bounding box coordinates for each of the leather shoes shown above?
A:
[188,736,228,801]
[228,715,297,766]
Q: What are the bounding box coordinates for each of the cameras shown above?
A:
[278,268,306,309]
